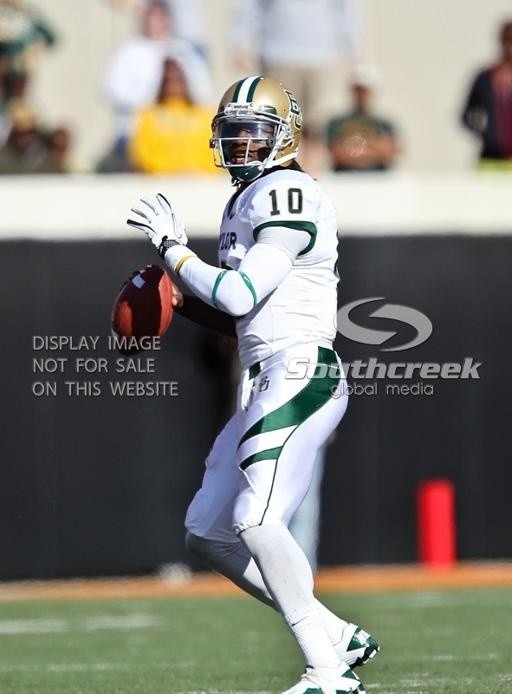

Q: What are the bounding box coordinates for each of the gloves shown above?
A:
[126,193,188,260]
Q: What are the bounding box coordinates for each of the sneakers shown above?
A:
[281,623,380,694]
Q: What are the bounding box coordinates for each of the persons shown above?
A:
[106,0,219,175]
[324,82,401,173]
[126,77,380,694]
[236,0,366,163]
[1,105,70,175]
[464,23,512,159]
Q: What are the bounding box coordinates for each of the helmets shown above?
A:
[209,75,304,167]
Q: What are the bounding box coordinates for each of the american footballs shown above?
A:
[111,265,173,356]
